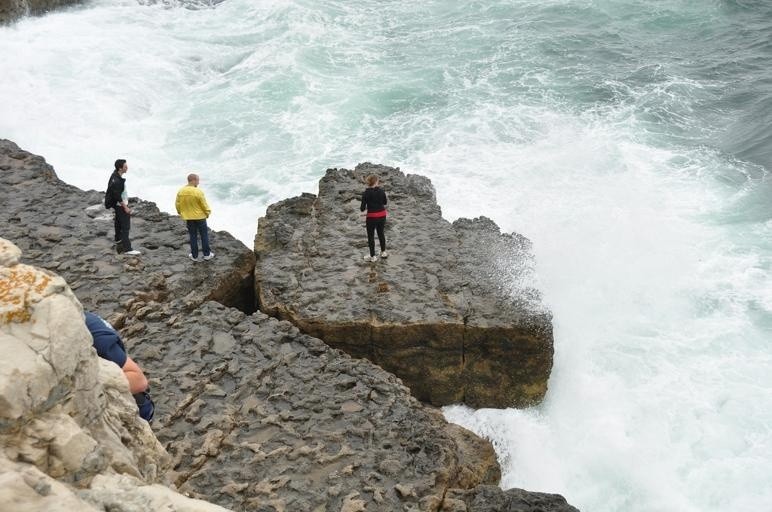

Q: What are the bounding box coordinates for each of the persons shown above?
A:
[104,158,143,256]
[84,309,156,425]
[174,173,215,264]
[358,172,390,264]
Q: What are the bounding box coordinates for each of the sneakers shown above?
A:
[124,250,141,255]
[381,251,388,258]
[363,254,378,262]
[188,253,198,262]
[203,251,215,261]
